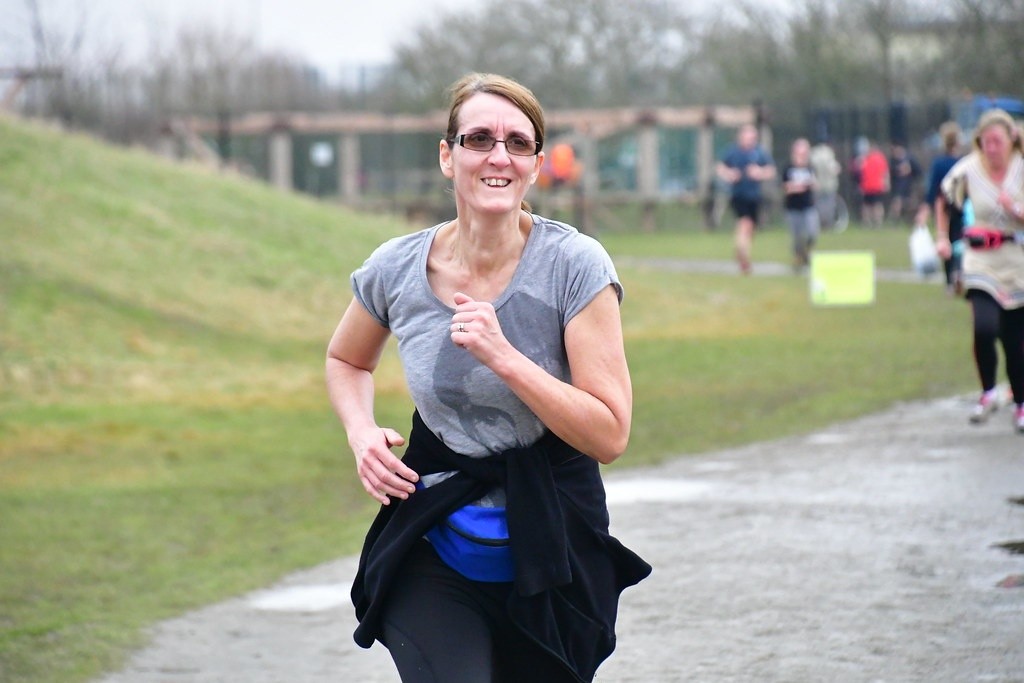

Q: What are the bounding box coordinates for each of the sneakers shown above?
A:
[968,394,999,424]
[1012,408,1024,433]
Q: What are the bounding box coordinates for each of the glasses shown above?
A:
[446,134,540,156]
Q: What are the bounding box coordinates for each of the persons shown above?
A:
[326,73,632,683]
[936,107,1023,436]
[714,120,964,299]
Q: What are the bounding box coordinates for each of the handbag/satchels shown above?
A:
[960,226,1016,250]
[909,224,942,279]
[418,481,515,583]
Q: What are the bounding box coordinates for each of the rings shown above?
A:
[458,323,466,332]
